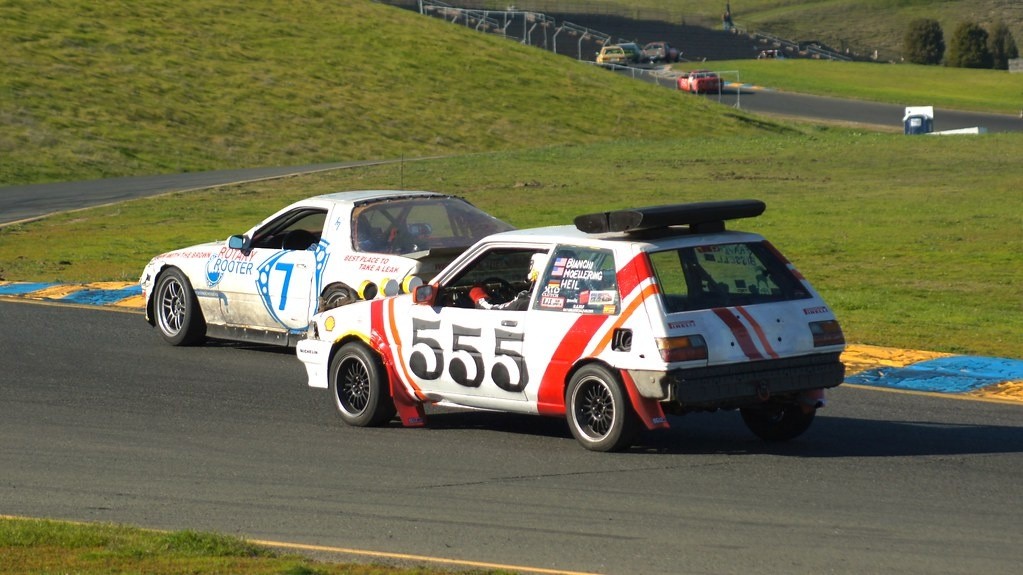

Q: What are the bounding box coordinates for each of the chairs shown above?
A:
[553,252,616,305]
[357,213,375,245]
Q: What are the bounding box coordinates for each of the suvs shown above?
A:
[294,198,847,454]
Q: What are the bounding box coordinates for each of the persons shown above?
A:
[469,252,549,311]
[721,11,732,30]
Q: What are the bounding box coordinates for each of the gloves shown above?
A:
[469,282,491,302]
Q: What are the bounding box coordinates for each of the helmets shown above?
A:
[527,253,547,283]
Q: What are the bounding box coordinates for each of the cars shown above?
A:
[616,41,681,64]
[677,69,724,94]
[137,187,520,351]
[594,46,627,64]
